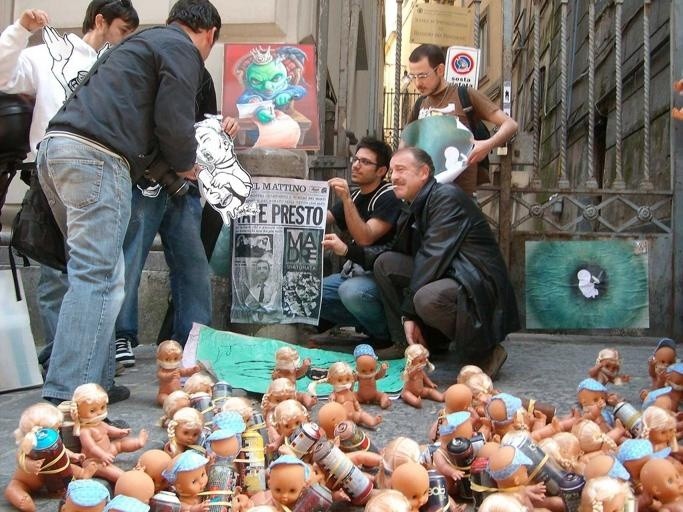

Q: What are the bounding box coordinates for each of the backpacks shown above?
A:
[412,86,490,172]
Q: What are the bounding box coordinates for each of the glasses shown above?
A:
[408,65,439,81]
[348,156,378,168]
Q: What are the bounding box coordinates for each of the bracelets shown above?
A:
[401,315,415,327]
[343,248,348,257]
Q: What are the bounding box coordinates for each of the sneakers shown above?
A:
[113,338,136,375]
[481,344,507,383]
[100,385,130,406]
[59,418,131,454]
[310,326,341,342]
[373,345,404,361]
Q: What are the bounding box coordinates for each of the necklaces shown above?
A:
[434,82,449,108]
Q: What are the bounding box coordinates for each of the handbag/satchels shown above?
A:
[12,173,65,272]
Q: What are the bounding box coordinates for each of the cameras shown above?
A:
[148,158,190,200]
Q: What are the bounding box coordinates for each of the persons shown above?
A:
[400,344,444,408]
[61,366,683,512]
[351,344,392,409]
[0,0,140,380]
[244,258,280,313]
[32,0,225,455]
[156,340,201,407]
[373,147,523,382]
[311,136,406,351]
[113,61,240,369]
[69,383,148,482]
[272,348,317,409]
[308,362,381,428]
[590,348,629,407]
[395,42,523,208]
[640,338,677,401]
[6,403,97,511]
